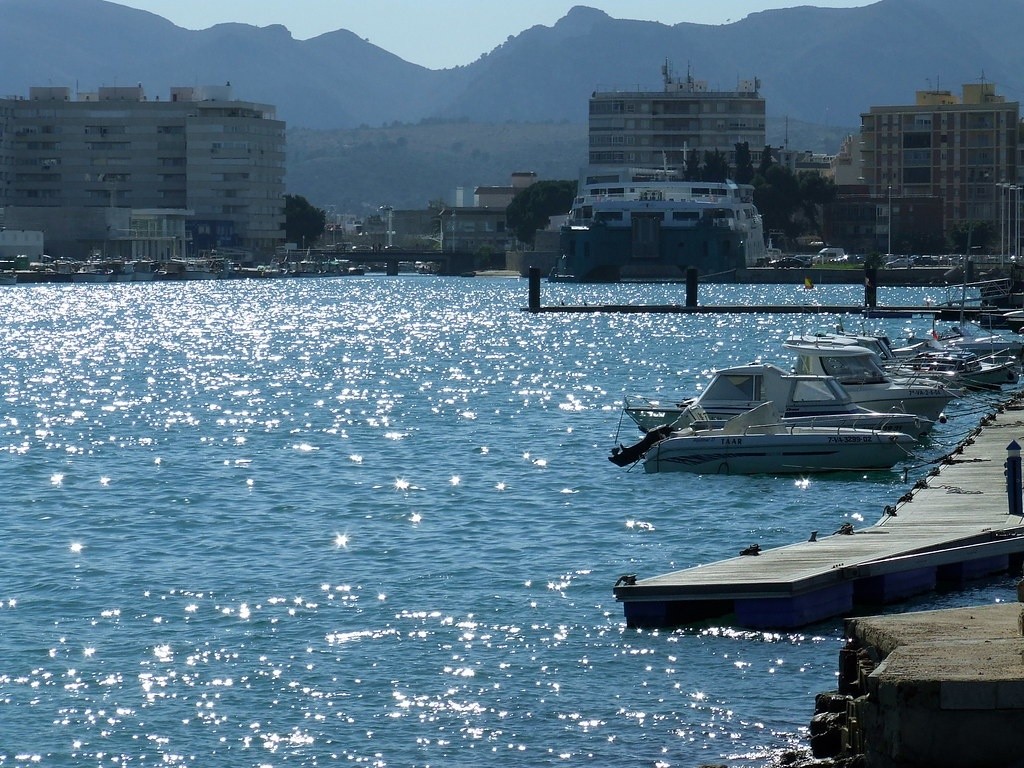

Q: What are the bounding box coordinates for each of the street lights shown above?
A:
[1006,185,1017,262]
[996,182,1010,268]
[1014,186,1023,256]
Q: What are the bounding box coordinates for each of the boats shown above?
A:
[783,311,1024,421]
[608,401,918,473]
[0,253,436,285]
[623,360,937,439]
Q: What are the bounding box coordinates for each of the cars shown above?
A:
[769,247,1007,269]
[336,242,399,251]
